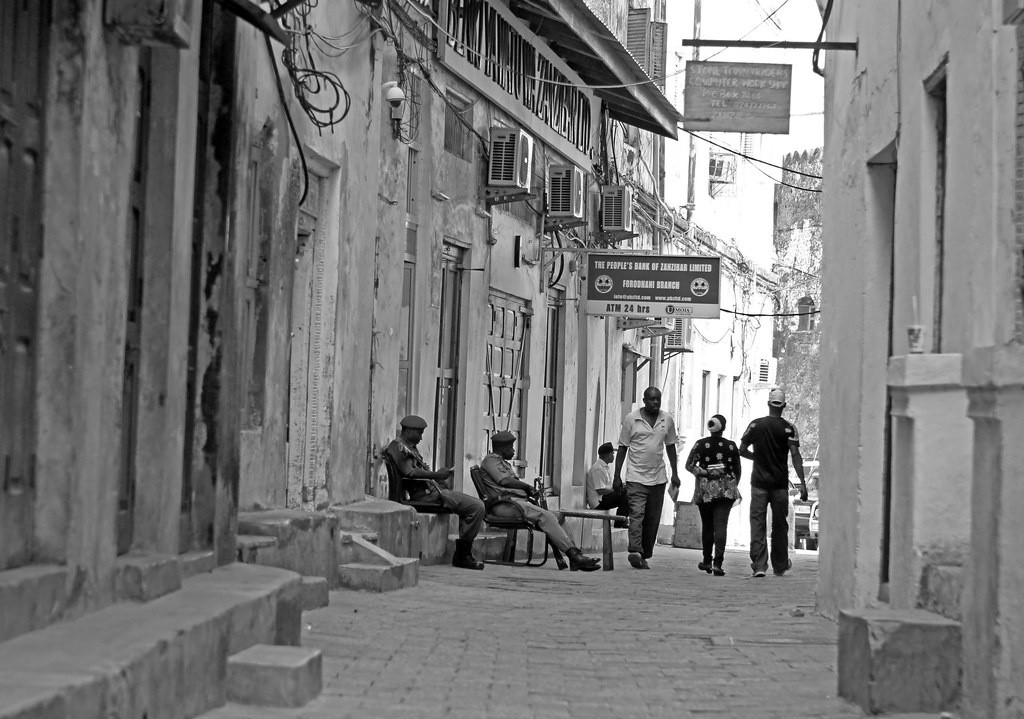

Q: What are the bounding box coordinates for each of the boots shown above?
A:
[567,546,602,571]
[451,540,484,571]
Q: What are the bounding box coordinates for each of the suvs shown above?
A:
[788,460,821,549]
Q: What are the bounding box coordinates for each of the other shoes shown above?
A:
[628,553,649,569]
[698,562,712,573]
[774,558,792,576]
[713,566,725,576]
[614,520,630,528]
[753,569,766,578]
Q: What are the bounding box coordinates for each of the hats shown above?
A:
[768,388,786,408]
[599,442,619,456]
[491,431,516,446]
[401,415,427,432]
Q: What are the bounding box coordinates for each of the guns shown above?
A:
[537,476,569,570]
[398,441,449,489]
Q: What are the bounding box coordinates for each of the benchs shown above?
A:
[547,508,627,570]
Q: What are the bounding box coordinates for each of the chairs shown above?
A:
[470,464,551,568]
[385,454,460,515]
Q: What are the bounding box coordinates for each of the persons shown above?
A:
[685,415,742,576]
[388,416,484,570]
[587,442,630,529]
[613,387,681,569]
[480,430,601,572]
[740,390,809,577]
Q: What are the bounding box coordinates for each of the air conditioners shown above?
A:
[547,163,584,220]
[664,318,696,348]
[758,356,778,383]
[105,0,192,48]
[711,157,732,181]
[645,318,675,331]
[487,123,535,194]
[602,185,634,232]
